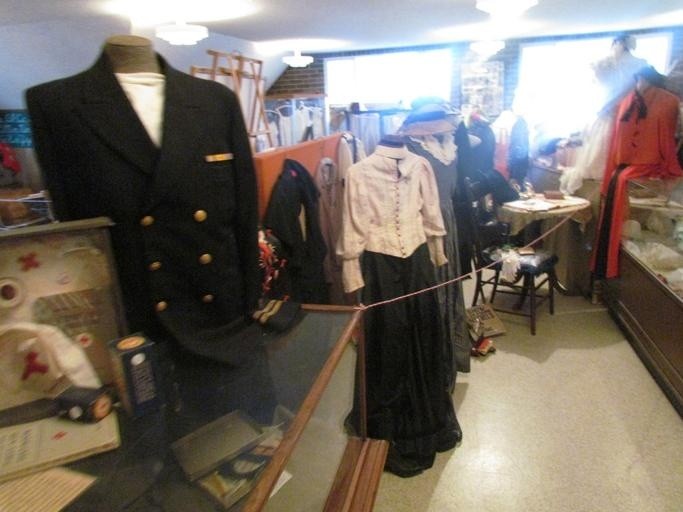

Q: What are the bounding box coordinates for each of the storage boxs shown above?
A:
[1,182,41,229]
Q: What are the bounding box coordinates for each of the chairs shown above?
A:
[462,172,558,335]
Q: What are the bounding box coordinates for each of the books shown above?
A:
[0,407,122,482]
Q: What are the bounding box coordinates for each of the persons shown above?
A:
[22,34,266,359]
[598,72,680,191]
[395,102,472,390]
[259,157,332,307]
[337,136,465,477]
[566,34,667,182]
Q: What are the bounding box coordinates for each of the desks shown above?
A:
[1,304,388,512]
[495,190,591,316]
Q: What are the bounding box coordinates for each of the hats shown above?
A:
[403,104,454,137]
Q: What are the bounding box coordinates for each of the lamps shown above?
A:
[155,15,210,47]
[283,48,315,68]
